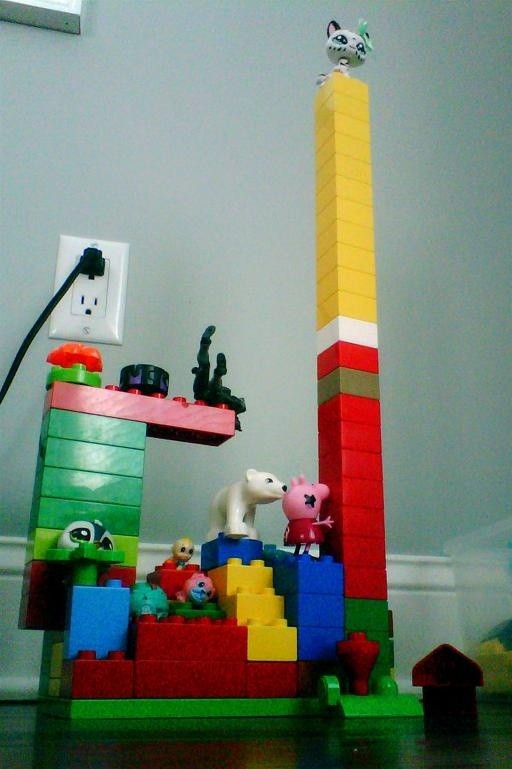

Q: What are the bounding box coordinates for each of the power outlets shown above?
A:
[48,234,130,346]
